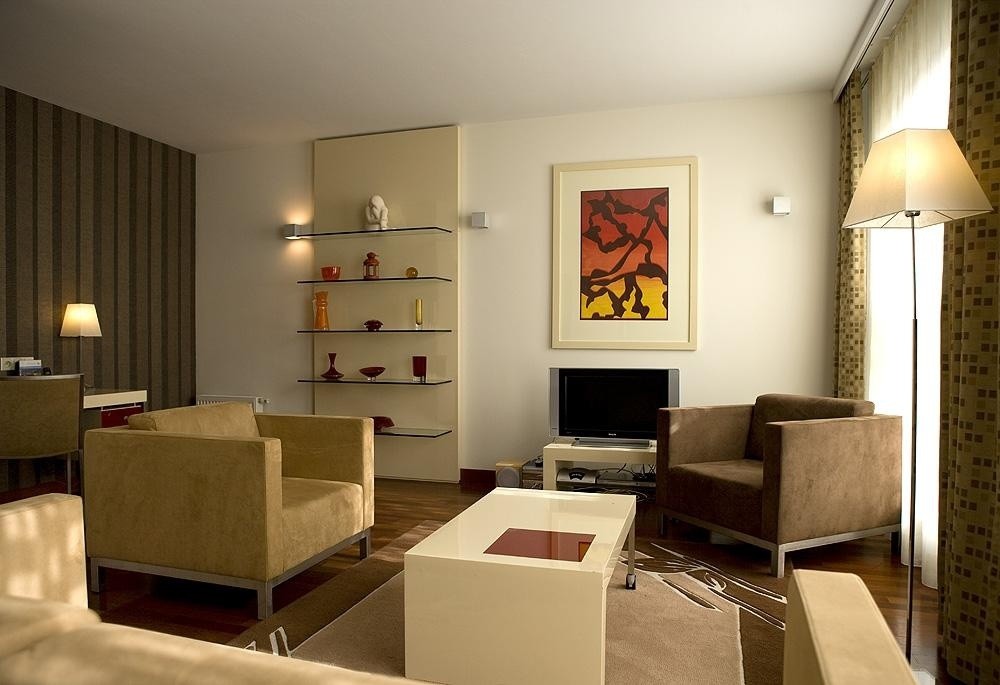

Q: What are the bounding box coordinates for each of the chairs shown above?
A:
[81,399,378,620]
[658,387,906,578]
[0,372,86,496]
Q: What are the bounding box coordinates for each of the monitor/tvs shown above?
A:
[548,368,680,450]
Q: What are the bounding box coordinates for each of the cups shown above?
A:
[412,356,427,383]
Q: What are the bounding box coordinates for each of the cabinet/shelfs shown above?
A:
[292,223,461,441]
[541,441,657,499]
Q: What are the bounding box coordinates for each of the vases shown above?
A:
[282,218,302,244]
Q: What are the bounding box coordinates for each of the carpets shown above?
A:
[228,515,799,685]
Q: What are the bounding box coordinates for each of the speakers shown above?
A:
[496,459,530,488]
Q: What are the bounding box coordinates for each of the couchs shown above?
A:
[782,566,924,685]
[0,488,421,685]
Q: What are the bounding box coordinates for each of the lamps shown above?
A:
[768,193,793,219]
[834,124,994,685]
[58,301,107,374]
[468,208,491,234]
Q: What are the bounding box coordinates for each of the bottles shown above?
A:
[312,291,329,330]
[320,353,344,379]
[320,266,341,281]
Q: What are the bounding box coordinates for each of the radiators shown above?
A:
[195,393,272,415]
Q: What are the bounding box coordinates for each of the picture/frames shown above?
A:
[545,151,703,355]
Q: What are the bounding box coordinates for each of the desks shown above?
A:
[398,482,642,685]
[81,386,148,428]
